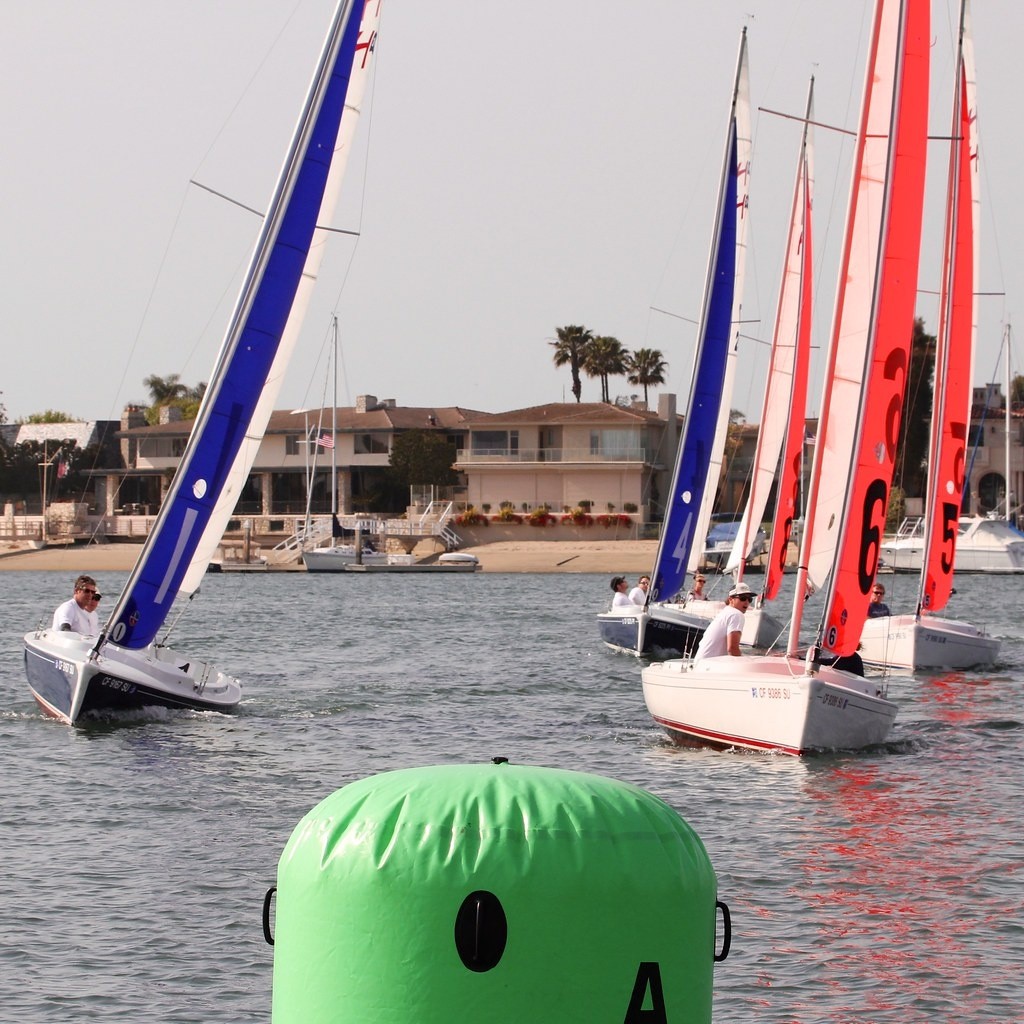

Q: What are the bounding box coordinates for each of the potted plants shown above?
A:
[455,499,639,514]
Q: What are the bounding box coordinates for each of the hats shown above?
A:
[610,575,625,590]
[95,587,102,598]
[729,583,758,597]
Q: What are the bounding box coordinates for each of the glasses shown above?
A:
[92,596,100,601]
[732,596,753,603]
[77,588,96,594]
[871,590,884,596]
[640,582,649,585]
[695,579,706,584]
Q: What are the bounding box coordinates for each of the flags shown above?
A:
[57,452,70,479]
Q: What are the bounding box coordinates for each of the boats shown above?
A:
[702,510,768,569]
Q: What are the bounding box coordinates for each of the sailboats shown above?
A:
[18,0,373,729]
[595,1,1003,760]
[298,313,484,575]
[873,324,1023,576]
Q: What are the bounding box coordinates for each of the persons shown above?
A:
[628,575,650,605]
[52,575,96,636]
[693,582,758,659]
[610,576,636,606]
[866,583,892,619]
[687,573,711,601]
[84,587,103,636]
[796,639,864,677]
